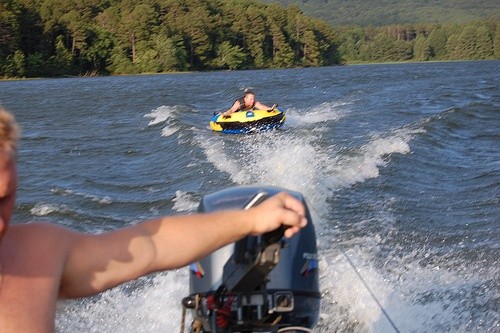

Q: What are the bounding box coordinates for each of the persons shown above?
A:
[219,92,276,116]
[0,108,309,333]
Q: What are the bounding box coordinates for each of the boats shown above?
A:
[209,106,287,136]
